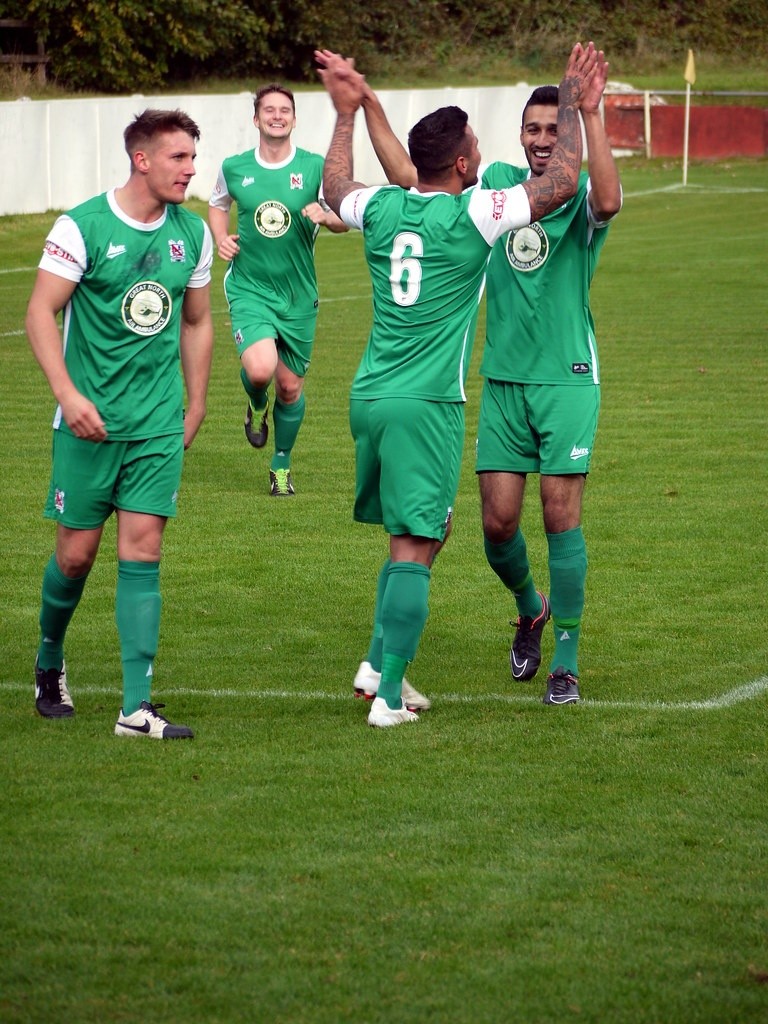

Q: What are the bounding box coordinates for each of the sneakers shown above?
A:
[543,666,580,705]
[244,392,269,448]
[509,591,550,681]
[35,653,75,718]
[270,469,295,495]
[115,700,194,739]
[368,697,419,727]
[353,662,431,710]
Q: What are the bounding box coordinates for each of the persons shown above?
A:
[313,41,622,725]
[23,111,216,742]
[210,84,352,495]
